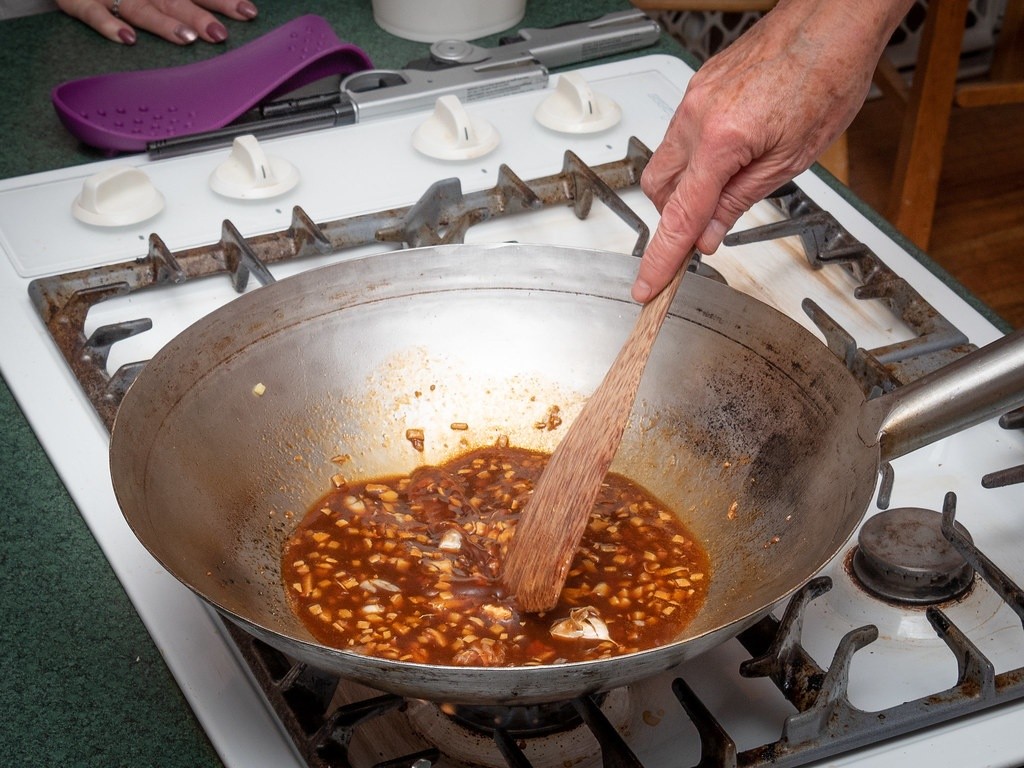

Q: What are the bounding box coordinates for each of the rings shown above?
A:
[111,0,121,17]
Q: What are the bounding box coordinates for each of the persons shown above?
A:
[56,0,259,46]
[631,0,915,302]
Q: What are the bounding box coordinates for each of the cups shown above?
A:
[371,1,526,43]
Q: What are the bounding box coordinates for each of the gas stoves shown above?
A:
[0,52,1024,768]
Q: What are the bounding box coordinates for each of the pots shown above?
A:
[107,244,1022,700]
